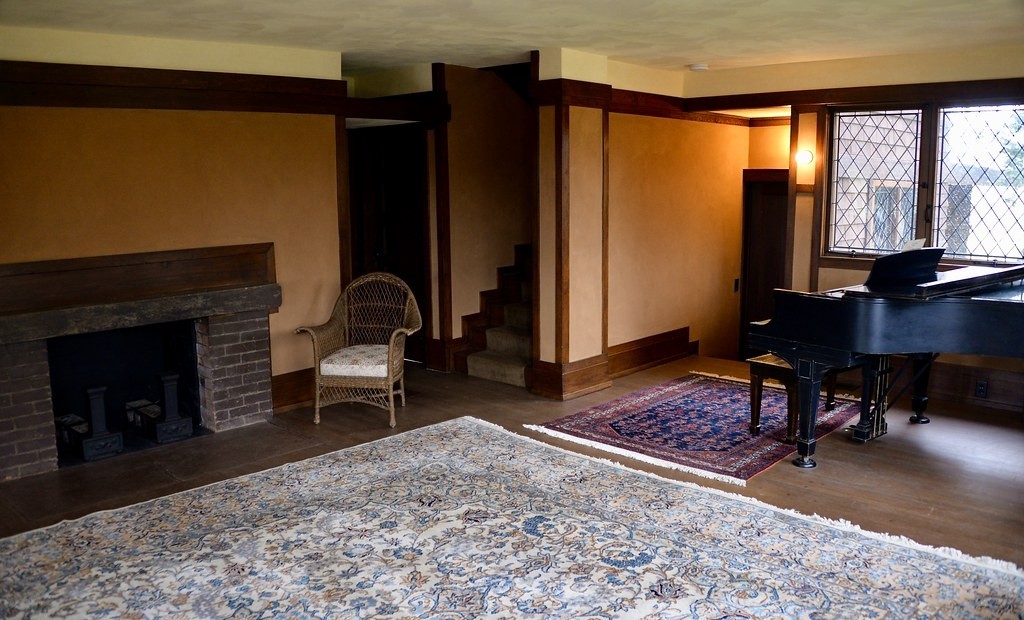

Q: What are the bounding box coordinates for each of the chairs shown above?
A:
[293,272,422,427]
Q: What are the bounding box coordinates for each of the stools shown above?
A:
[745,353,838,445]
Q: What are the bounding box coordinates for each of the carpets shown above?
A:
[522,371,888,487]
[0,415,1024,620]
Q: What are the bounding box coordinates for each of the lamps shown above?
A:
[795,151,813,164]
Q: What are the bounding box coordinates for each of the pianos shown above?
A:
[746,246,1024,469]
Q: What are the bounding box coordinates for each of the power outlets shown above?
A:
[976,380,988,398]
